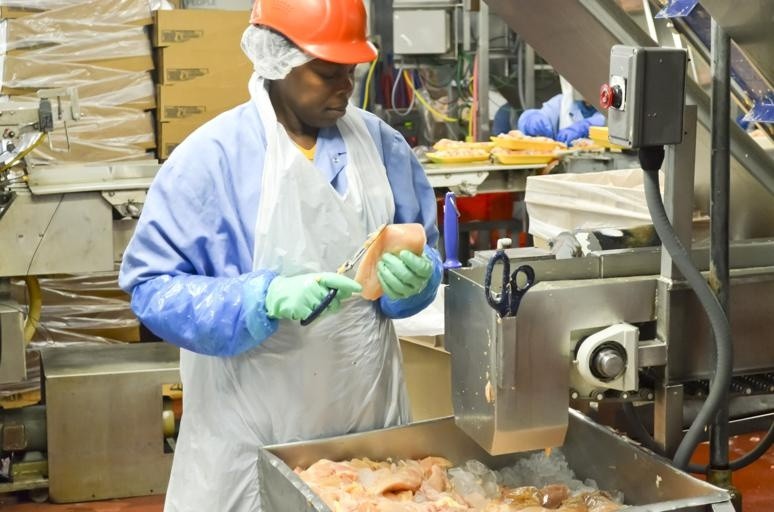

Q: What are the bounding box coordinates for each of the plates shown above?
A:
[425,132,568,165]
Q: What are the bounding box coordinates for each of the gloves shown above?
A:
[525,112,553,138]
[556,121,592,147]
[376,250,434,301]
[265,271,362,325]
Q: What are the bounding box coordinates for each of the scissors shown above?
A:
[484,248,536,390]
[299,221,388,326]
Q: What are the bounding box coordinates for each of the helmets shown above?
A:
[248,1,379,65]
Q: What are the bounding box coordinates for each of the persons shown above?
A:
[120,1,442,512]
[517,93,605,143]
